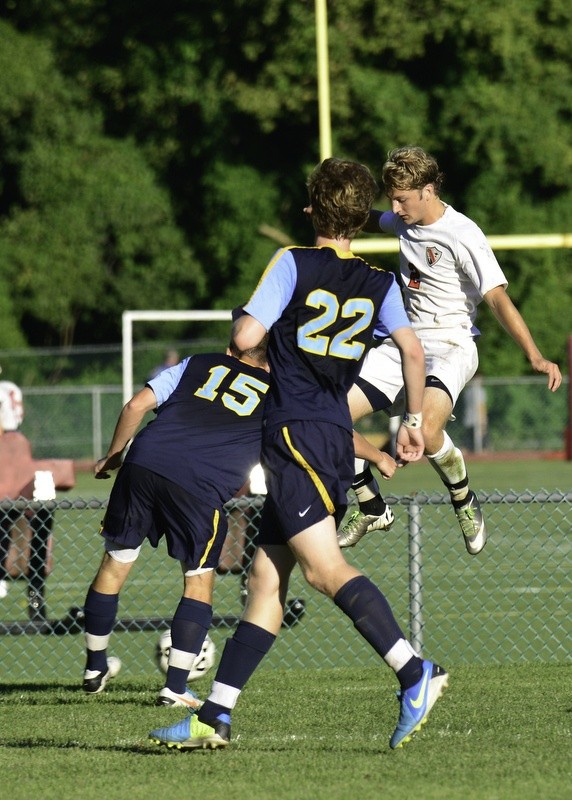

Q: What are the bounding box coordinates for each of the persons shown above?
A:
[300,147,562,555]
[148,156,450,751]
[0,156,450,751]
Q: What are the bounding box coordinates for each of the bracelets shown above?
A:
[399,411,421,433]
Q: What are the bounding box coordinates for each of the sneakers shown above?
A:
[82,656,121,694]
[337,504,395,547]
[155,686,205,709]
[453,491,487,555]
[389,659,449,749]
[148,705,232,750]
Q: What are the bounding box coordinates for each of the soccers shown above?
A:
[155,627,217,682]
